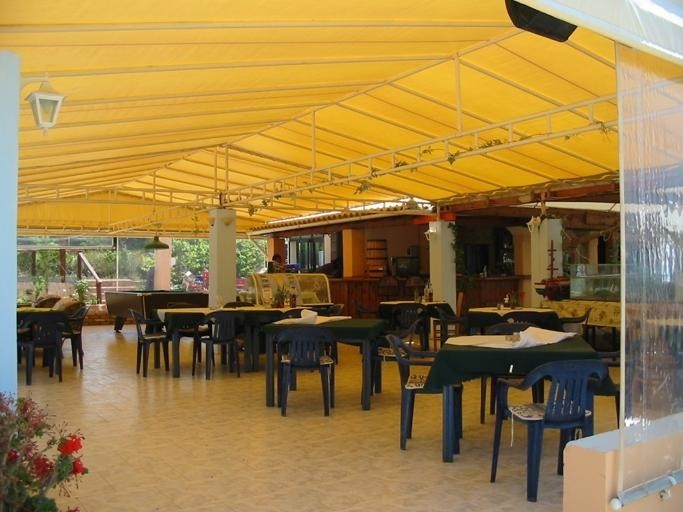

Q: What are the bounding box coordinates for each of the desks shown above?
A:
[103,289,209,335]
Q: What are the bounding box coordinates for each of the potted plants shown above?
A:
[271,284,289,308]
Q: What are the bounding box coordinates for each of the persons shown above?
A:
[272,254,288,270]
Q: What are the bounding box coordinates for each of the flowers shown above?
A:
[0,391,92,512]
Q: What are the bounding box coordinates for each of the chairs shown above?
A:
[17,292,93,388]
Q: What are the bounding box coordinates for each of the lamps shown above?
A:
[525,214,542,233]
[143,234,170,251]
[19,74,68,135]
[423,226,437,241]
